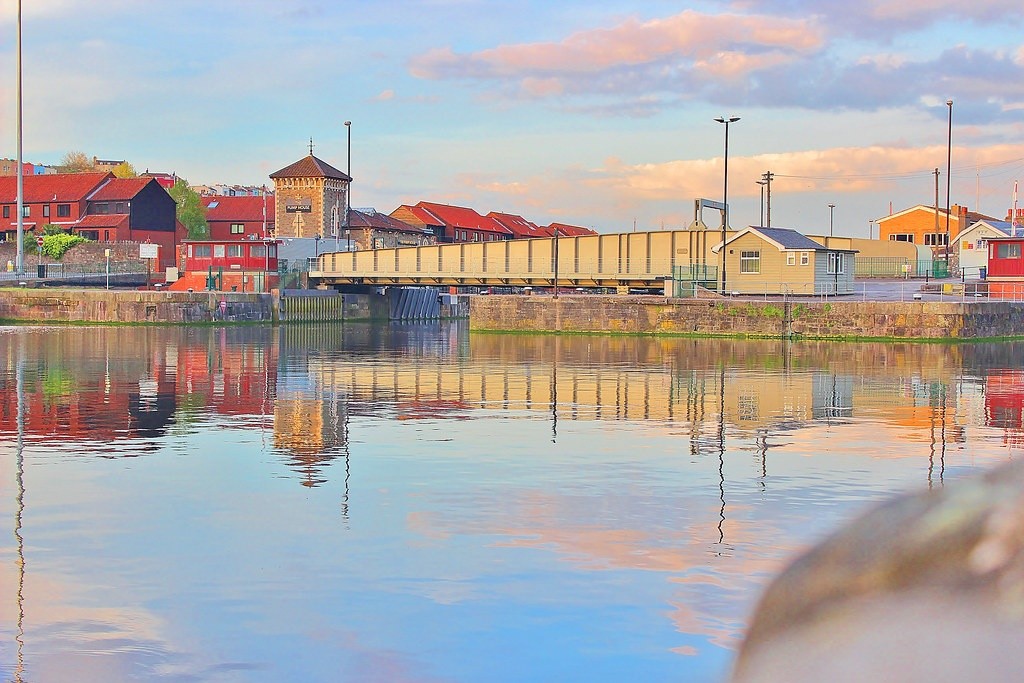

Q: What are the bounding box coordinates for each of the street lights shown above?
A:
[344,120,352,251]
[714,118,741,295]
[947,99,953,265]
